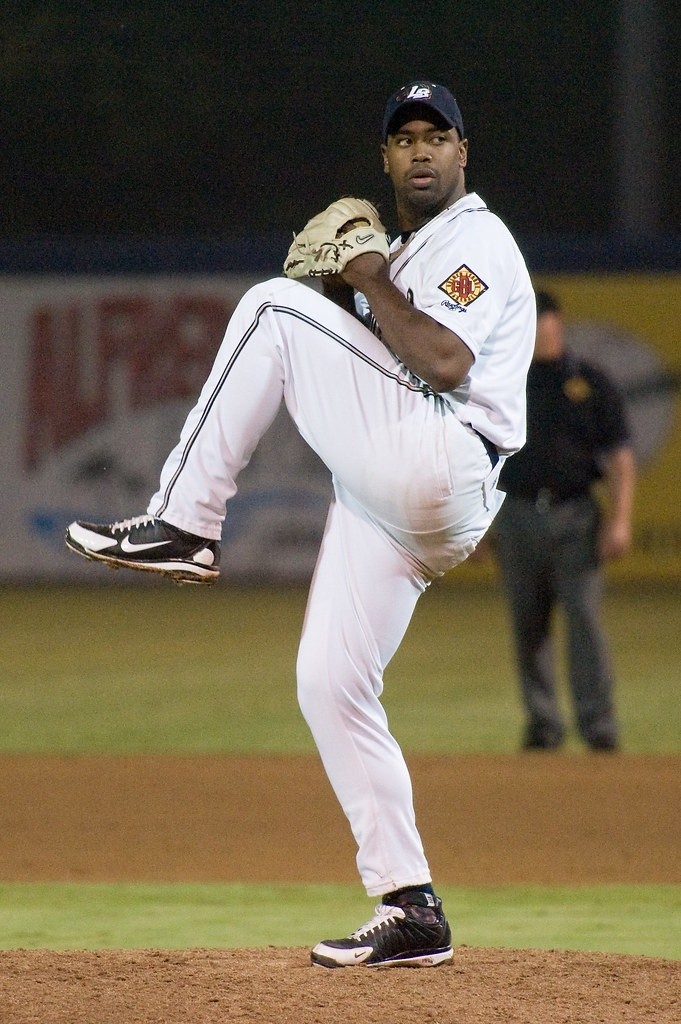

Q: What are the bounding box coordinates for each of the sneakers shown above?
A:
[311,898,456,971]
[65,514,220,585]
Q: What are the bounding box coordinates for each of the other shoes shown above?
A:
[523,725,566,750]
[581,721,620,749]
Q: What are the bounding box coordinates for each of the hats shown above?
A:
[381,79,465,145]
[534,289,559,318]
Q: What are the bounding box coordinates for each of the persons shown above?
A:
[65,82,539,970]
[470,289,647,755]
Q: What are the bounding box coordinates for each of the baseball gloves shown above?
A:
[282,195,390,280]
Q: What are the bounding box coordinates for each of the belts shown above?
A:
[471,425,507,485]
